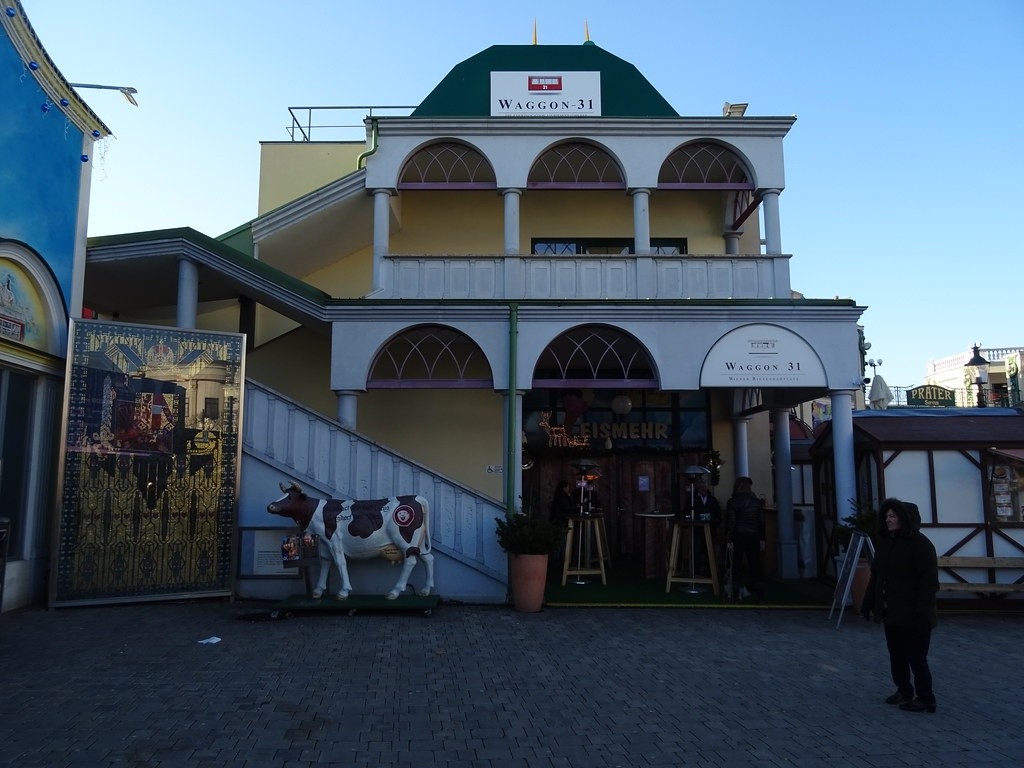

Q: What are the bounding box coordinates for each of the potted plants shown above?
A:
[831,510,872,610]
[491,507,573,614]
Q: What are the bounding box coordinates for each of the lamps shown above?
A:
[727,103,749,118]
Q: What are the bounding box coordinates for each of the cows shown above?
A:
[267,480,434,602]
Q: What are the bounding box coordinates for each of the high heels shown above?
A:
[899,694,936,713]
[885,688,915,705]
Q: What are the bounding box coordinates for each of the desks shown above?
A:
[560,513,607,585]
[581,512,613,568]
[662,519,721,597]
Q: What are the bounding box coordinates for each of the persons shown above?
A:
[688,479,722,591]
[862,497,940,713]
[552,482,570,506]
[726,477,766,599]
